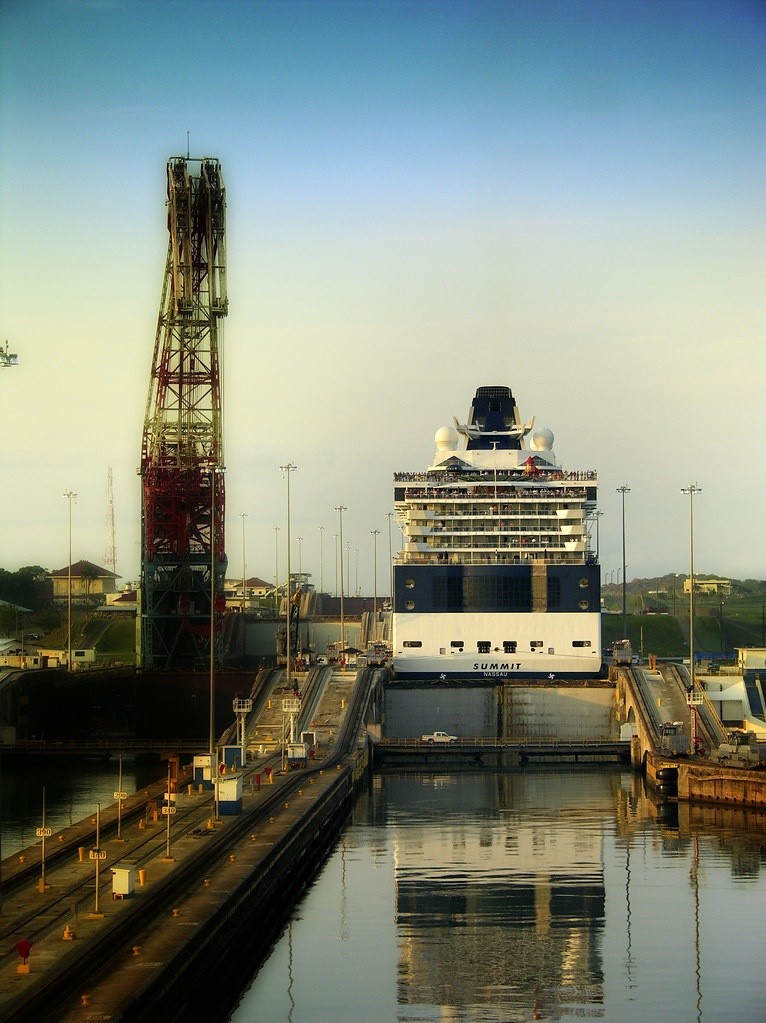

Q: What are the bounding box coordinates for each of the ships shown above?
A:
[385,381,606,682]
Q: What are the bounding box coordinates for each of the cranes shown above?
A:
[126,128,233,747]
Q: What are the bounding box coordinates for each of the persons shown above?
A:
[392,470,596,565]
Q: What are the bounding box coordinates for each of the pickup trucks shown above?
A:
[421,730,458,745]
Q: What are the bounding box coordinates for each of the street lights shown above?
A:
[693,572,699,617]
[673,574,680,616]
[278,460,299,681]
[719,601,725,653]
[272,526,281,619]
[616,483,631,638]
[296,537,303,586]
[332,535,339,596]
[202,460,227,755]
[238,512,248,612]
[369,529,381,641]
[384,512,394,610]
[333,504,348,661]
[681,484,705,755]
[656,579,662,614]
[60,490,77,670]
[317,526,324,594]
